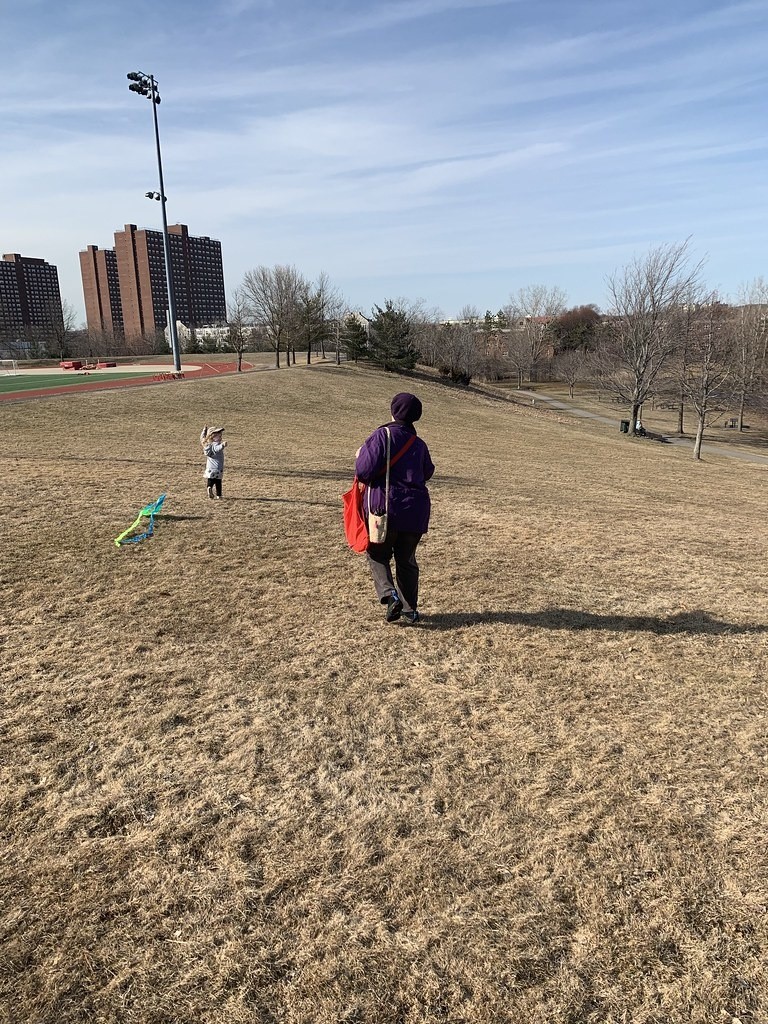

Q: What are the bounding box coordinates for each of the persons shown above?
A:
[200,424,229,499]
[354,392,435,624]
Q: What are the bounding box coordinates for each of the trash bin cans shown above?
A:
[620,419,630,433]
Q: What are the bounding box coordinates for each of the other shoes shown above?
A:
[405,610,419,625]
[216,496,220,499]
[386,590,403,621]
[207,486,214,499]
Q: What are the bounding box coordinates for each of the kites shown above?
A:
[115,494,167,548]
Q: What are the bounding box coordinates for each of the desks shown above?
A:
[612,396,623,404]
[730,418,738,428]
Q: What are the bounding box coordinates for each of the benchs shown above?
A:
[655,404,674,409]
[611,398,632,404]
[724,421,728,428]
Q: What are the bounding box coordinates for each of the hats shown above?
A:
[207,427,225,436]
[391,392,422,426]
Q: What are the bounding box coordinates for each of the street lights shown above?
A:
[127,70,181,373]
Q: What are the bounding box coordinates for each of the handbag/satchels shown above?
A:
[341,474,369,553]
[368,512,388,544]
